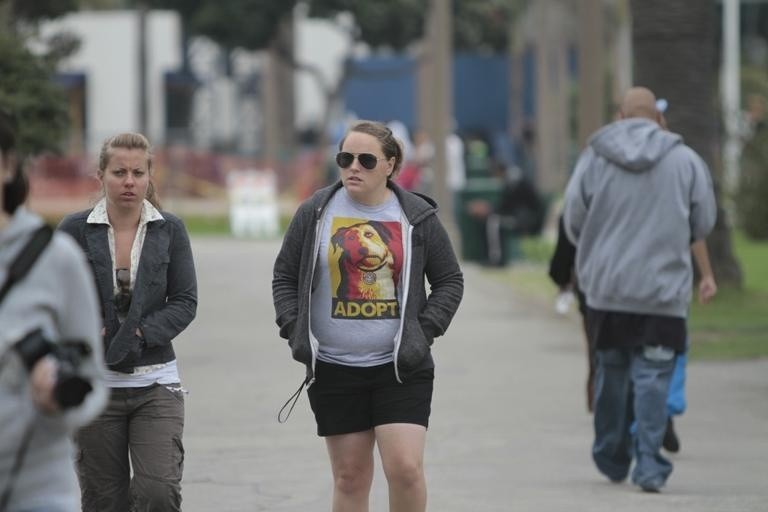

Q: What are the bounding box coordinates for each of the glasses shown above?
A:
[335,152,388,169]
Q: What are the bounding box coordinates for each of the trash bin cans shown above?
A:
[452,189,514,266]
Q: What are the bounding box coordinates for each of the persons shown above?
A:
[546,86,722,493]
[1,105,110,511]
[250,117,545,271]
[271,119,465,511]
[54,131,201,511]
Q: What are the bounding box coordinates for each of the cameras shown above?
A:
[13,328,93,408]
